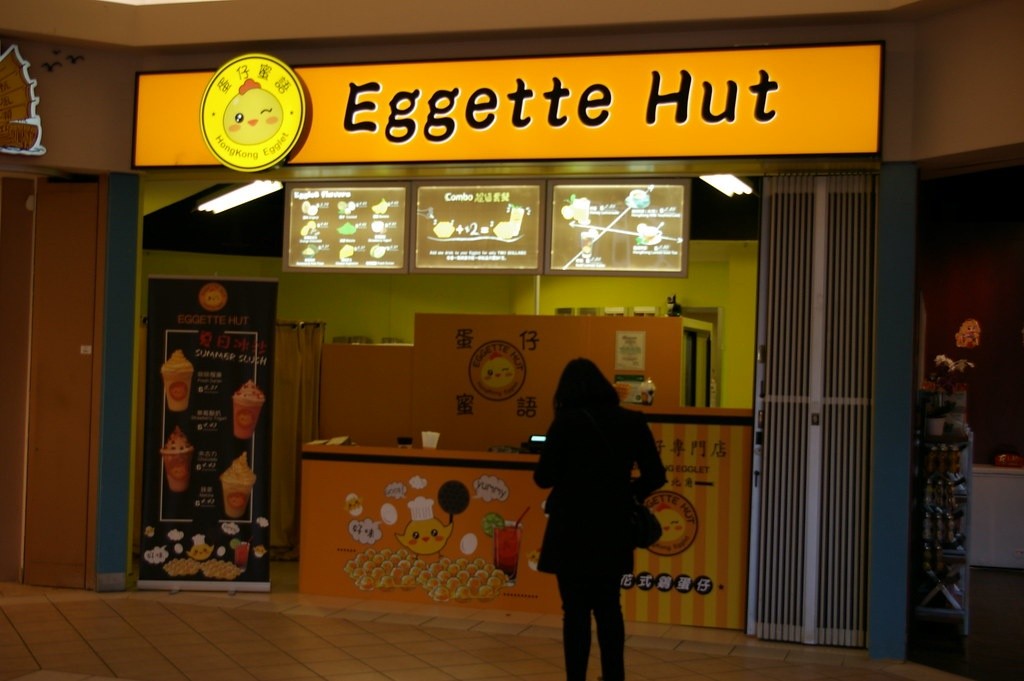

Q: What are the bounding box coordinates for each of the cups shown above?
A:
[234,542,250,572]
[493,527,522,580]
[220,476,256,519]
[232,398,266,441]
[160,444,195,493]
[161,368,194,412]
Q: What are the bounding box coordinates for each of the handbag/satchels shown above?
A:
[630,487,663,548]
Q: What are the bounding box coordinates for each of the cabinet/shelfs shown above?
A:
[914,430,974,636]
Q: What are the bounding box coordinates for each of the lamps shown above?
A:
[699,174,754,197]
[197,181,284,215]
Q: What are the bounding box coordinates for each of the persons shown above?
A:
[531,357,669,681]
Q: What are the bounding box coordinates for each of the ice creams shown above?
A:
[232,379,265,439]
[161,349,194,411]
[220,451,257,518]
[160,426,195,492]
